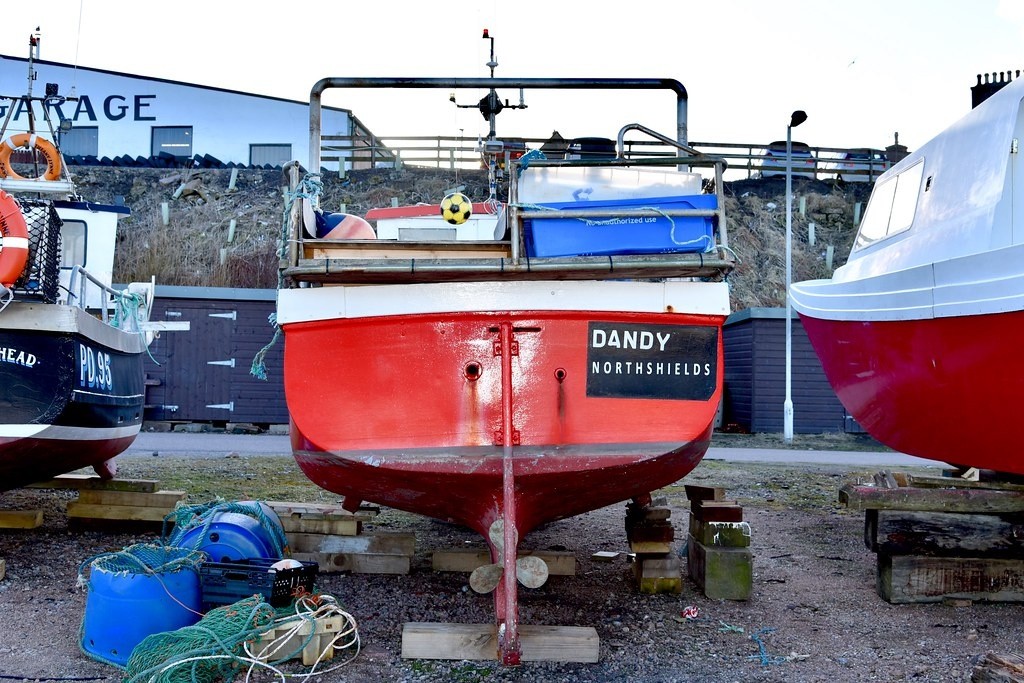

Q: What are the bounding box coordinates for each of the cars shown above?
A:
[751,140,815,180]
[481,141,526,170]
[823,148,890,182]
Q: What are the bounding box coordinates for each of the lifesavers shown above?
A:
[0,188,29,299]
[0,133,63,181]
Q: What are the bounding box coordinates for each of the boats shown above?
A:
[0,25,191,492]
[789,71,1024,475]
[276,28,743,666]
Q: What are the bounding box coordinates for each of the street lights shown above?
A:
[784,110,807,442]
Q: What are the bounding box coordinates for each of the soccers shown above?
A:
[440,192,473,225]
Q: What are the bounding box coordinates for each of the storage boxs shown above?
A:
[198,558,343,669]
[508,167,719,259]
